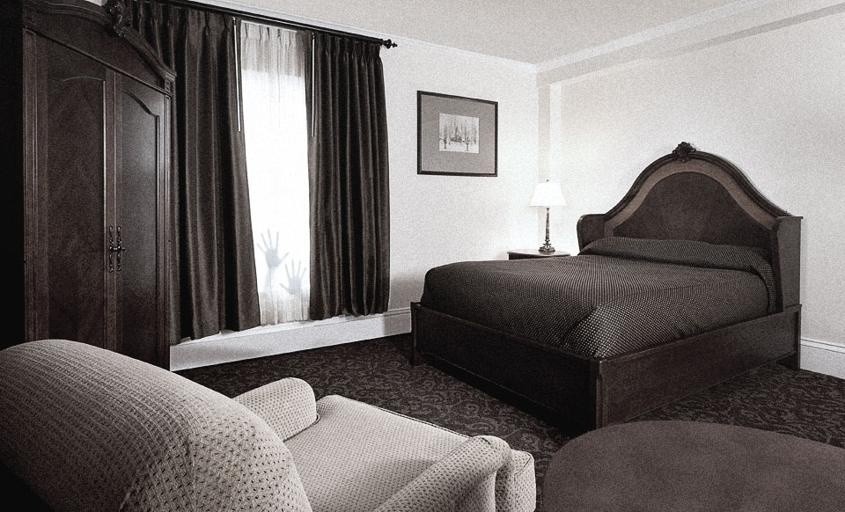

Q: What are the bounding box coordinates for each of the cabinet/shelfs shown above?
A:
[0,0,177,360]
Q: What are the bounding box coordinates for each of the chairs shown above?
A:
[0,340,538,512]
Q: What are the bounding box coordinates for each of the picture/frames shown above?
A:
[416,90,499,177]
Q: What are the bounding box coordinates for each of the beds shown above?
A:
[411,142,800,424]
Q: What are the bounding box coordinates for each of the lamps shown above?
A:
[530,177,568,254]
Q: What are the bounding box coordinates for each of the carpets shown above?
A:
[540,421,845,512]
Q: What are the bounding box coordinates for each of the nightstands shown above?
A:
[508,248,568,258]
